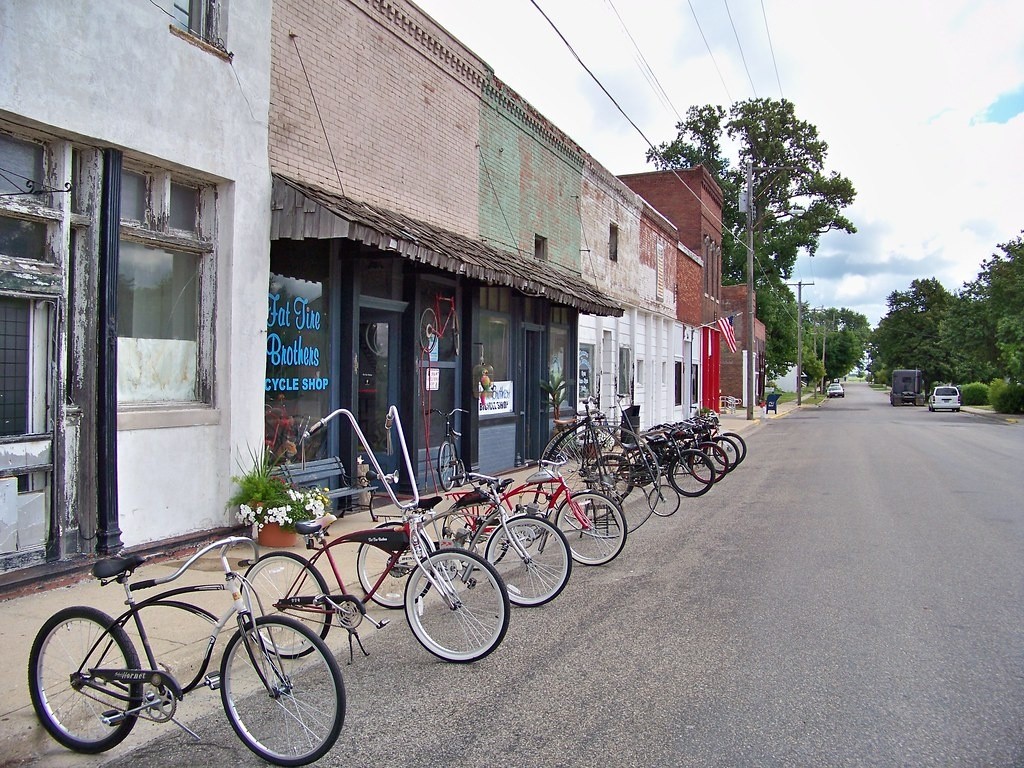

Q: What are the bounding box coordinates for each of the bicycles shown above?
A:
[421,405,467,491]
[27,535,347,768]
[240,405,512,664]
[356,393,747,611]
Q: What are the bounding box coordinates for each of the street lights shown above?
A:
[746,209,807,422]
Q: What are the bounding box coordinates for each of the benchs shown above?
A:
[270,456,379,523]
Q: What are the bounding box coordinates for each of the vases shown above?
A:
[251,502,296,547]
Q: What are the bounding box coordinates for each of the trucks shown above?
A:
[889,369,924,407]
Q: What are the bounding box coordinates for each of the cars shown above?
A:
[826,383,845,399]
[927,386,961,413]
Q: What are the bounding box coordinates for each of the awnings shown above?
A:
[271,173,626,318]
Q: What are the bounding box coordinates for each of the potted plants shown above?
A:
[582,429,614,460]
[539,366,569,437]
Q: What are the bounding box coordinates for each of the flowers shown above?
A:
[223,437,332,540]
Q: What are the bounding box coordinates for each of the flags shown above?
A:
[717,315,737,353]
